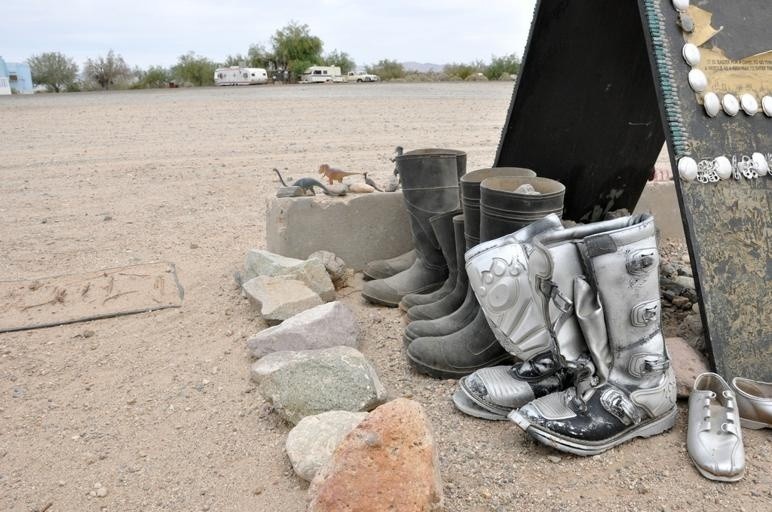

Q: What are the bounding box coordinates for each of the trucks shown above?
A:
[212,61,268,85]
[302,64,342,84]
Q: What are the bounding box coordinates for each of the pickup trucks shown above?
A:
[340,71,365,84]
[358,72,377,82]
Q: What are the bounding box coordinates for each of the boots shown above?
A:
[399,208,463,314]
[506,213,678,457]
[402,167,537,347]
[360,153,460,308]
[404,214,464,327]
[452,213,596,421]
[406,177,567,380]
[363,149,466,282]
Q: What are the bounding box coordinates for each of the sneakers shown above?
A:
[686,372,746,482]
[732,375,771,431]
